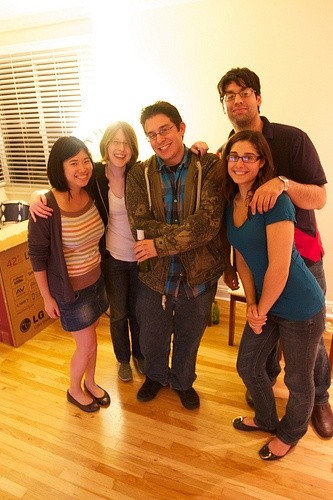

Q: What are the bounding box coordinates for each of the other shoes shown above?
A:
[132,355,144,374]
[311,402,333,438]
[245,387,255,408]
[176,386,200,409]
[84,380,110,405]
[258,437,295,461]
[67,388,100,412]
[232,416,263,431]
[137,375,163,402]
[117,360,132,382]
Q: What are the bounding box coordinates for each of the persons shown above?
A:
[29,121,209,381]
[221,131,326,460]
[27,136,111,414]
[216,68,333,438]
[124,102,224,409]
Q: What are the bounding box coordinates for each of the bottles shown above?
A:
[212,301,219,324]
[136,226,150,273]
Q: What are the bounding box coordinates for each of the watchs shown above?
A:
[279,176,289,191]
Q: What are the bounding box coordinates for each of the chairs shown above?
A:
[227,245,247,346]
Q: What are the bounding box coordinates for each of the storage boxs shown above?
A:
[0,219,58,345]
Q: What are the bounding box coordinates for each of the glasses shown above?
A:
[146,123,174,142]
[226,154,262,164]
[221,88,256,102]
[111,139,129,147]
